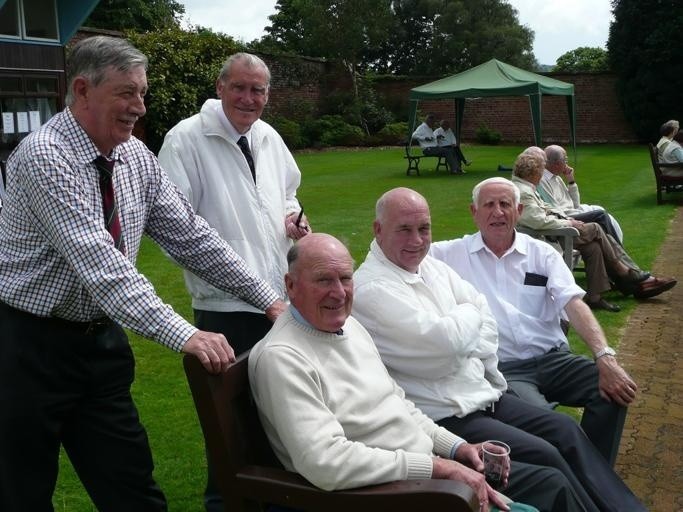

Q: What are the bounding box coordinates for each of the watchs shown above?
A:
[594,347,616,361]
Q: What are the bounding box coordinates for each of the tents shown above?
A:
[407,55,578,164]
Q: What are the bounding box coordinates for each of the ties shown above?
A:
[235,135,256,184]
[94,156,125,258]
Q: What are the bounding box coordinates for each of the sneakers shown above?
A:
[465,160,473,166]
[461,169,467,173]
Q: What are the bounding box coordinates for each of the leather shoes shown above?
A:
[635,275,677,297]
[586,298,620,312]
[613,268,650,288]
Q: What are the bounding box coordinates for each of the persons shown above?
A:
[411,114,458,174]
[433,119,473,173]
[0,37,289,511]
[523,147,677,300]
[156,52,311,511]
[510,152,650,319]
[656,120,683,179]
[248,232,537,512]
[348,187,646,511]
[544,144,624,246]
[427,177,637,471]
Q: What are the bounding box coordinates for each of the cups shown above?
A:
[482,440,511,490]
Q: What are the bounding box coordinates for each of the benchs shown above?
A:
[648,142,683,206]
[404,136,449,176]
[183,348,480,512]
[517,227,581,270]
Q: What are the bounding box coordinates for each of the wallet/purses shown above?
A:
[524,272,548,288]
